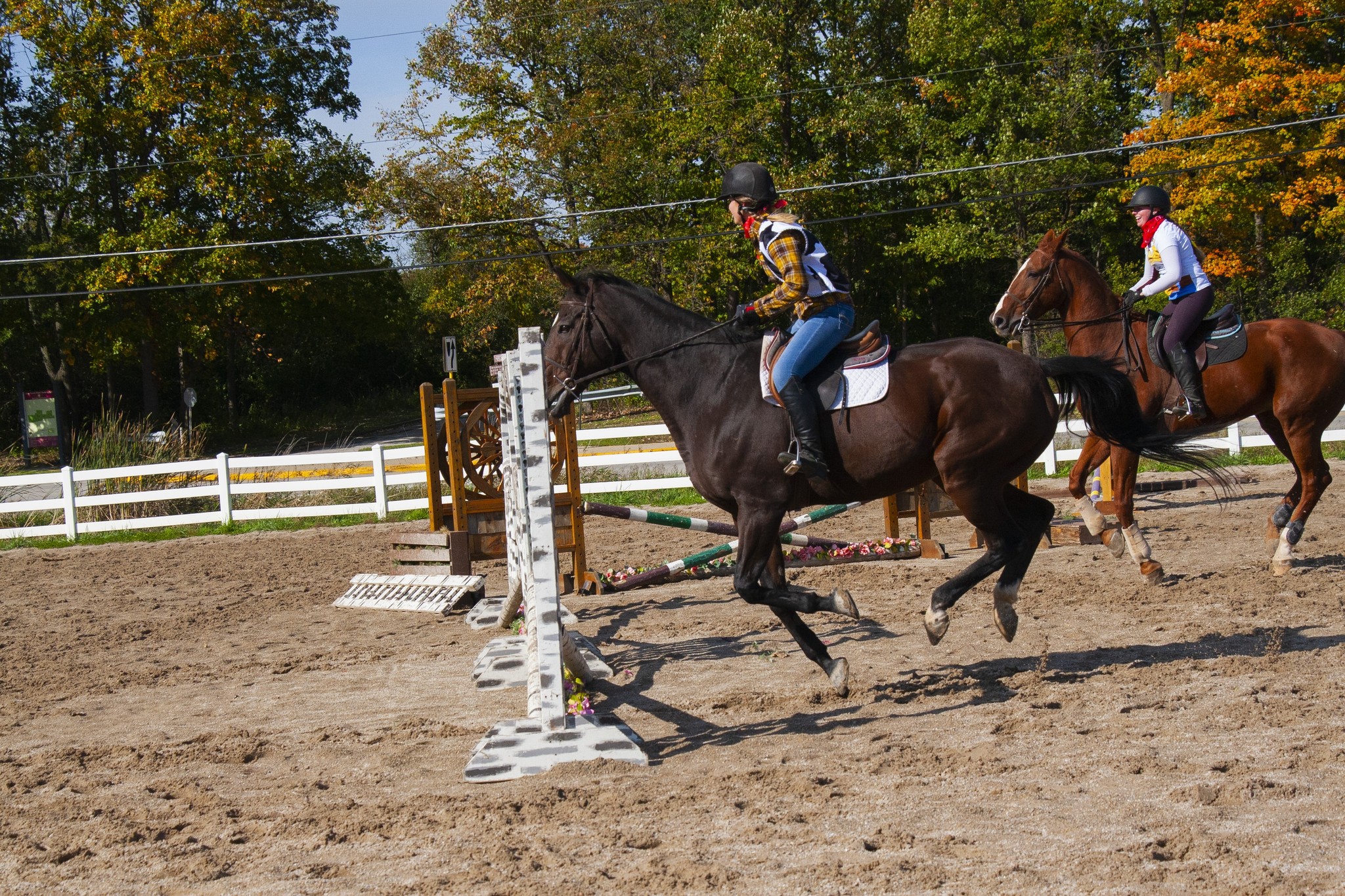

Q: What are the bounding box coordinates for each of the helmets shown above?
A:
[1124,185,1170,214]
[716,162,777,201]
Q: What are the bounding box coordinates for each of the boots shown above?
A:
[777,375,828,479]
[1167,342,1207,420]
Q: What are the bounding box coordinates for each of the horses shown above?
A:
[990,228,1345,585]
[543,265,1258,696]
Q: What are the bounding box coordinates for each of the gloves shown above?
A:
[735,301,761,330]
[1125,289,1146,307]
[1122,290,1133,299]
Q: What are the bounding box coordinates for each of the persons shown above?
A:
[1121,185,1215,424]
[716,163,857,482]
